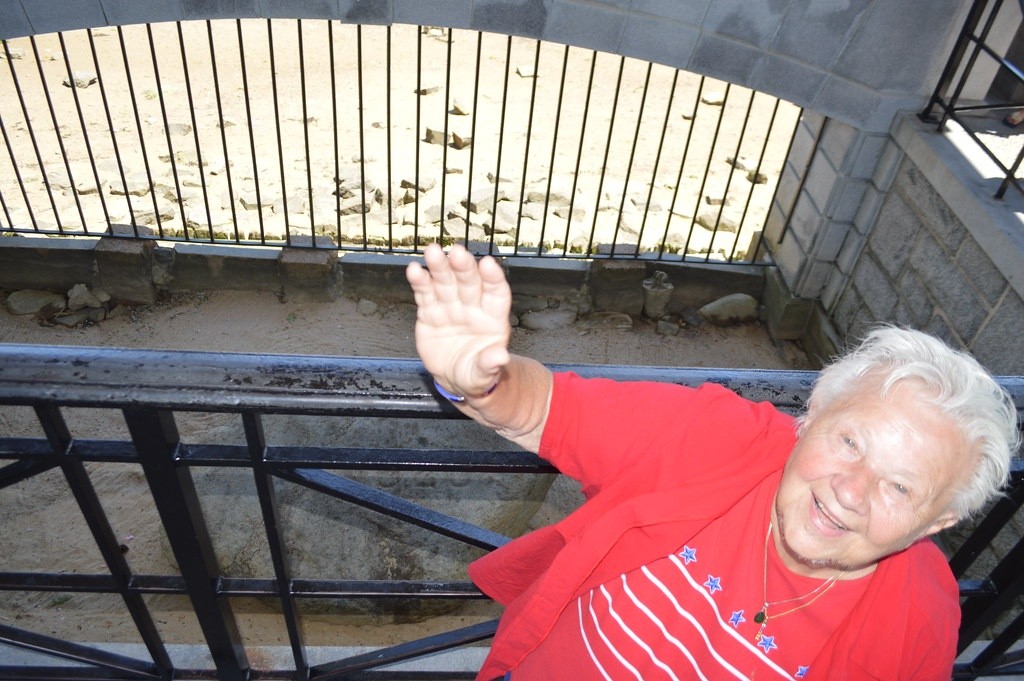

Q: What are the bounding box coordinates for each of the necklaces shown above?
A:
[754,522,848,641]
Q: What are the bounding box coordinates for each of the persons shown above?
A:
[404,242,1024,681]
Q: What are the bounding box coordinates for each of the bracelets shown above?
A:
[433,370,503,403]
